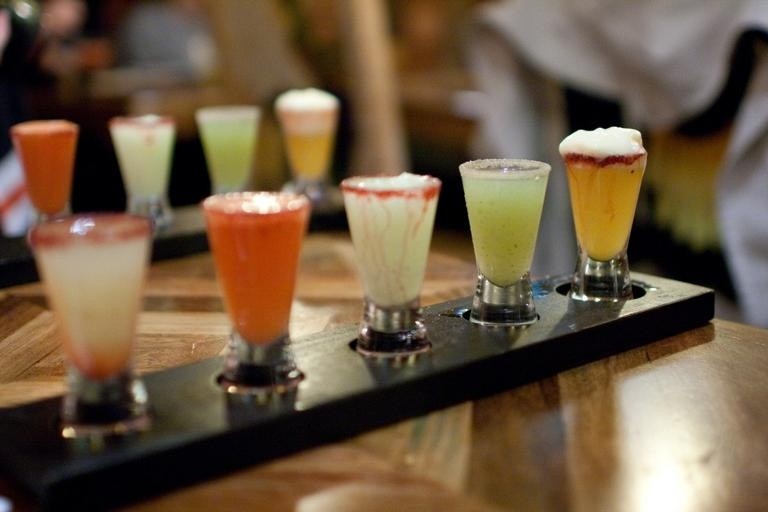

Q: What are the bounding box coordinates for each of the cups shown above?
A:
[272,88,342,202]
[195,104,260,191]
[8,120,80,223]
[25,213,158,436]
[110,113,177,226]
[339,173,444,353]
[202,192,313,391]
[560,127,648,302]
[458,157,552,325]
